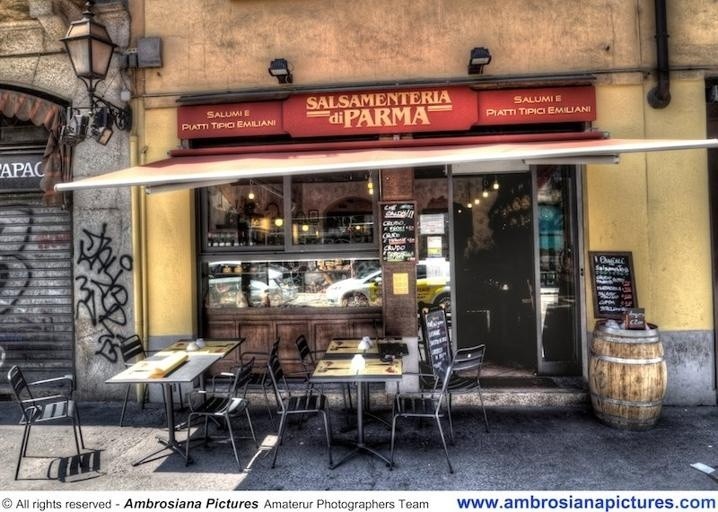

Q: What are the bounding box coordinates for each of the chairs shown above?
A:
[7,364,86,481]
[389,299,490,474]
[184,333,335,469]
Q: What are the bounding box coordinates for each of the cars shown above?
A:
[327,261,452,312]
[204,263,296,306]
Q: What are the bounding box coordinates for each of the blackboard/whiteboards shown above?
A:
[588,252,639,321]
[375,202,420,264]
[423,308,453,384]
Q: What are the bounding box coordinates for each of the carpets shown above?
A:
[420,375,559,390]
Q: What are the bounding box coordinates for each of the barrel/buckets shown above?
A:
[588,320,668,432]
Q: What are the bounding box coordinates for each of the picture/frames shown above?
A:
[308,209,319,223]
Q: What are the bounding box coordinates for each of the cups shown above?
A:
[351,353,366,370]
[185,338,206,351]
[356,336,373,350]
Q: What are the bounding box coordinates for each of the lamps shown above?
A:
[59,2,136,148]
[467,47,491,75]
[248,179,255,200]
[466,174,499,208]
[367,170,373,189]
[267,58,293,84]
